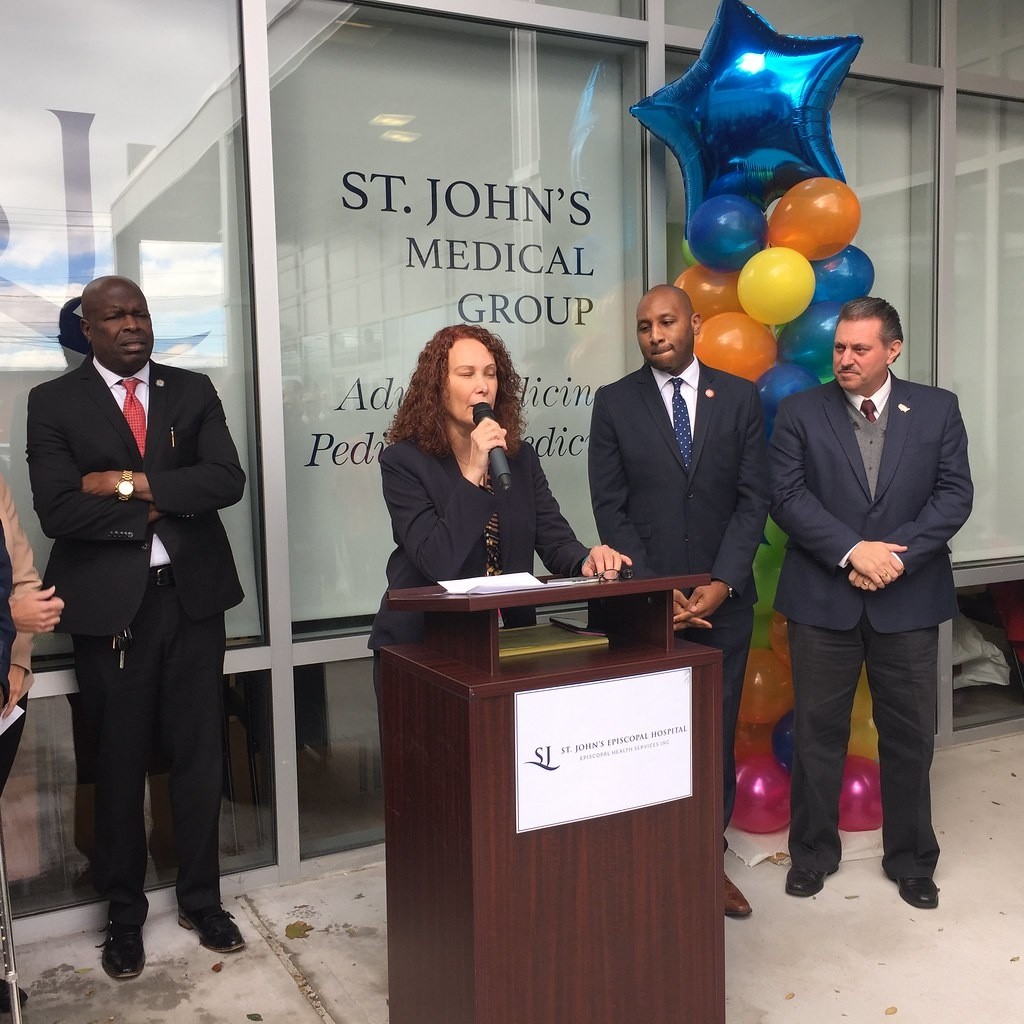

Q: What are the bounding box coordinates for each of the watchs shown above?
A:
[726,583,739,600]
[115,469,135,501]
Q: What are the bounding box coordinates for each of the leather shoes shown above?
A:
[96,920,146,978]
[786,864,839,897]
[177,905,245,953]
[897,876,939,908]
[723,872,752,915]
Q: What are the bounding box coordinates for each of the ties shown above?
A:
[860,400,876,423]
[670,378,692,472]
[117,378,146,459]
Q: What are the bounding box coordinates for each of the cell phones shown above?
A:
[549,616,606,636]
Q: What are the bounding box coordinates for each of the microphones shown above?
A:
[472,403,512,491]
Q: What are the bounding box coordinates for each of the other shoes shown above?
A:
[0,979,28,1009]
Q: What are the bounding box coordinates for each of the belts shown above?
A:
[147,565,176,586]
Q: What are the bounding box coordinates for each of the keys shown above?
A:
[118,638,128,668]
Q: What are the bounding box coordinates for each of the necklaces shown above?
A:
[457,457,468,465]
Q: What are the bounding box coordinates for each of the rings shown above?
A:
[862,580,869,586]
[882,573,889,580]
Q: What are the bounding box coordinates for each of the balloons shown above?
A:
[673,176,874,423]
[627,0,865,241]
[732,509,886,833]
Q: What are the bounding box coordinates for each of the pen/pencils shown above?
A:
[171,427,175,447]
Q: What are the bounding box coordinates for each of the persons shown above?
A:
[0,474,66,1011]
[365,321,633,698]
[587,286,771,917]
[763,297,975,908]
[28,276,247,980]
